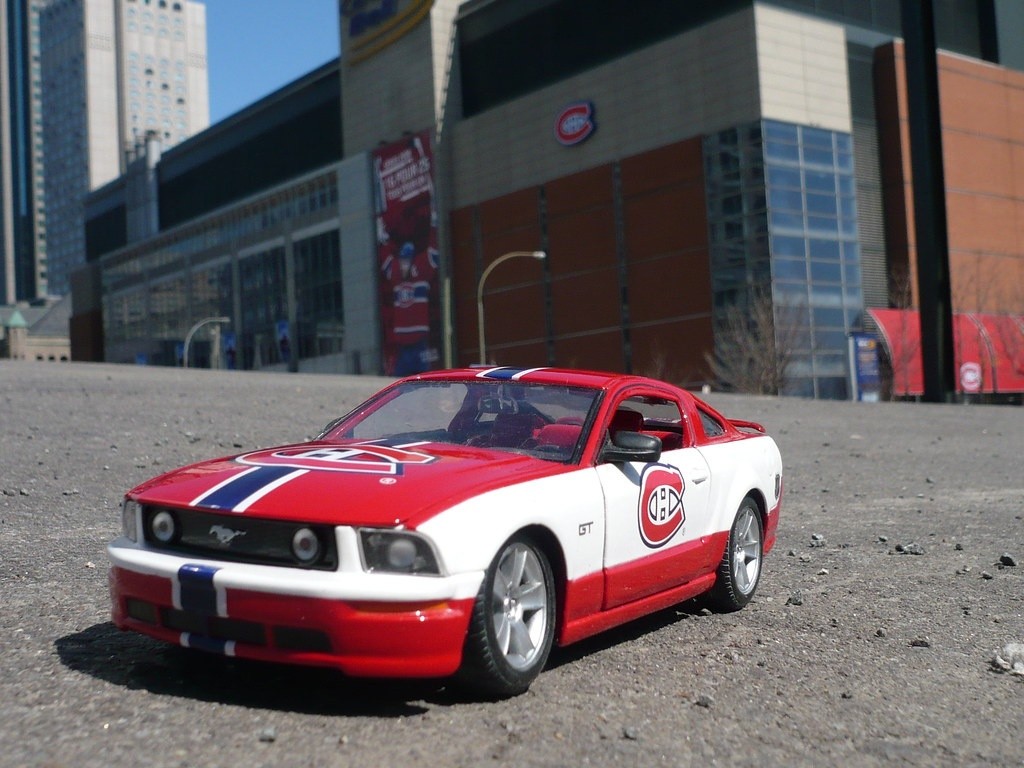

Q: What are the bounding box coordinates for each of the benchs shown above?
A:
[528,424,677,451]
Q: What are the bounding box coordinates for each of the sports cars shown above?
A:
[102,366,786,700]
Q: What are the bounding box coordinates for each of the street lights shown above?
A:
[477,249,549,364]
[181,318,231,368]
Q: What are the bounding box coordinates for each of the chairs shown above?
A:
[607,411,647,435]
[462,413,547,447]
